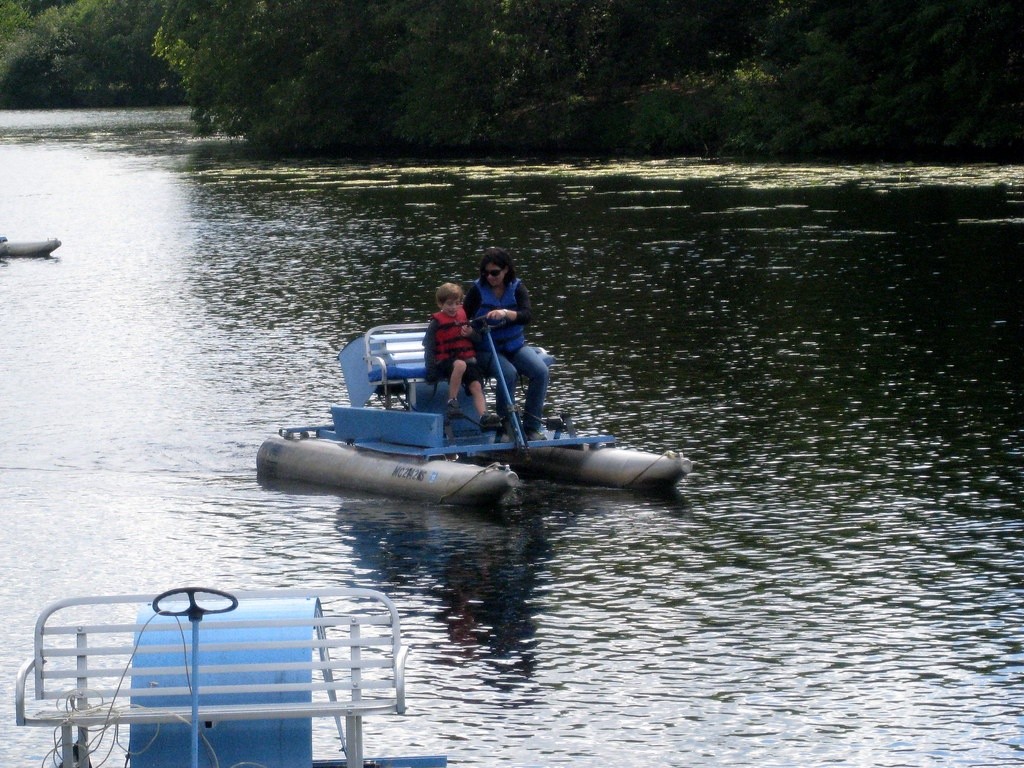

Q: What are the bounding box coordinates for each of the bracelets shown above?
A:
[503,307,509,319]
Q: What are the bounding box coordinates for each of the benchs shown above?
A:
[15,587,412,768]
[364,321,434,410]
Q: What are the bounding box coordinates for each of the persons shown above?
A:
[463,246,551,440]
[420,282,502,433]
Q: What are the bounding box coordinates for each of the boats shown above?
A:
[15,578,454,767]
[251,293,689,514]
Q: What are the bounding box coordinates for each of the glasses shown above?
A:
[481,268,504,276]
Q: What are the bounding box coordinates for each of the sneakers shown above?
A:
[478,414,501,428]
[523,429,547,442]
[504,423,528,441]
[447,398,463,416]
